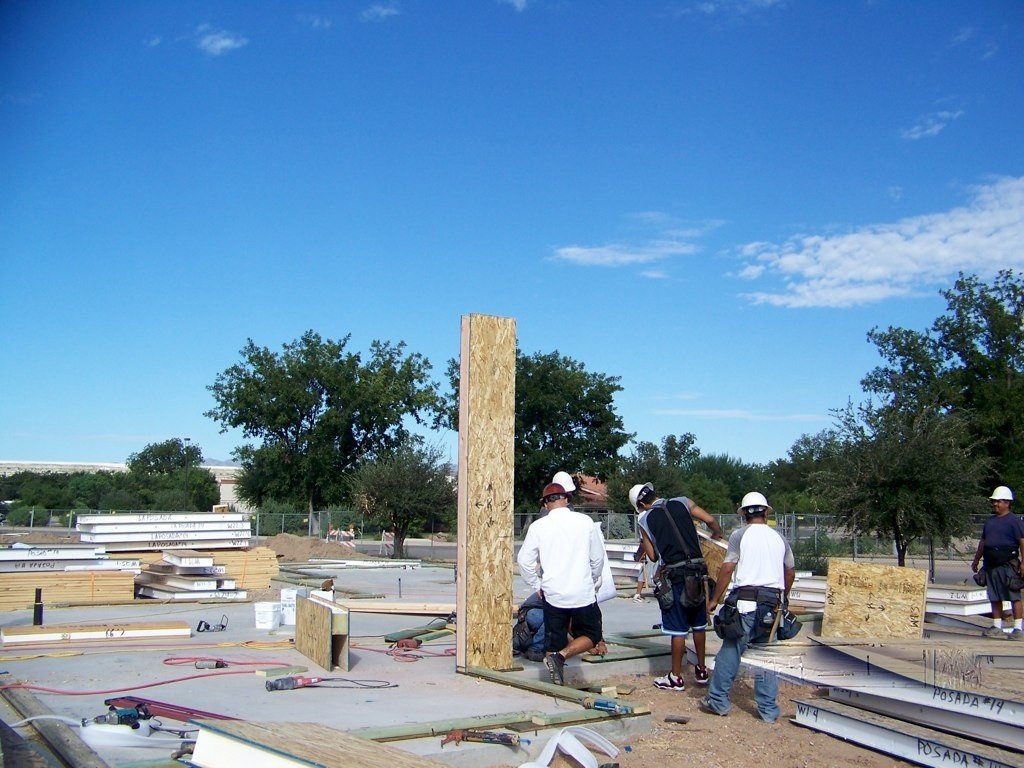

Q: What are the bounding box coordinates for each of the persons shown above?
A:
[628,481,725,692]
[970,486,1024,640]
[517,587,610,662]
[517,482,604,686]
[539,472,579,520]
[631,525,662,604]
[700,491,796,724]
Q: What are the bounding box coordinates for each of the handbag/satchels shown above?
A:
[1006,560,1024,591]
[713,593,804,639]
[652,562,717,611]
[634,541,647,563]
[513,617,534,654]
[973,565,987,588]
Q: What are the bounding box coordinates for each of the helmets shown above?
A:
[629,482,654,513]
[542,484,572,507]
[989,486,1015,501]
[737,492,772,516]
[552,471,576,493]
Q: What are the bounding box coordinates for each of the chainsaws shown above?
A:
[265,675,324,691]
[80,703,154,730]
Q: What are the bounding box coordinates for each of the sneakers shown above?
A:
[653,671,687,691]
[632,596,650,603]
[543,653,565,686]
[983,626,1003,636]
[1008,629,1023,640]
[694,664,709,685]
[701,696,729,716]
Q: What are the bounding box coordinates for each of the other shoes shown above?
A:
[525,650,545,662]
